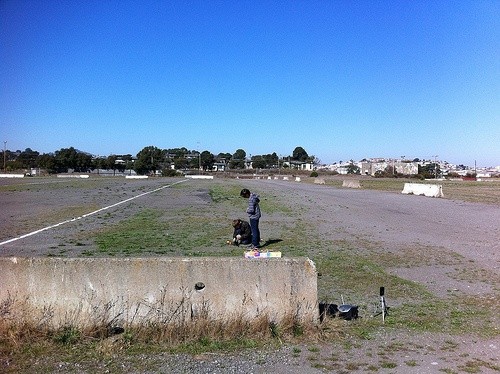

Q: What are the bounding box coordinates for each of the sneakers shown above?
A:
[247,243,258,250]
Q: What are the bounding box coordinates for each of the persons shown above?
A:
[240,189,262,251]
[232,219,253,246]
